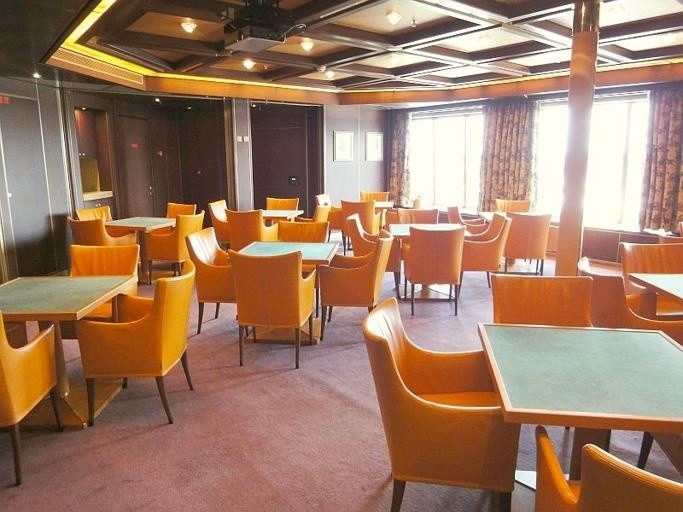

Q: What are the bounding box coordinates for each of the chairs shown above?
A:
[227,247,316,370]
[402,225,466,316]
[456,218,511,297]
[395,207,440,286]
[446,205,487,233]
[576,256,682,346]
[489,274,593,327]
[66,190,403,333]
[463,213,505,242]
[490,199,532,213]
[362,298,522,512]
[618,240,681,321]
[72,258,197,426]
[1,309,63,485]
[412,199,420,209]
[503,211,552,276]
[534,425,681,510]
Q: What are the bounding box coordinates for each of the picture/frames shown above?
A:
[365,131,384,162]
[333,129,354,163]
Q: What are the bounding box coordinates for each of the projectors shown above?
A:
[224,25,286,53]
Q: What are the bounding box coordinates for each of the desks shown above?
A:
[477,320,681,510]
[626,270,680,305]
[2,275,138,428]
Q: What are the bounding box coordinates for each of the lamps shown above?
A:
[176,1,430,82]
